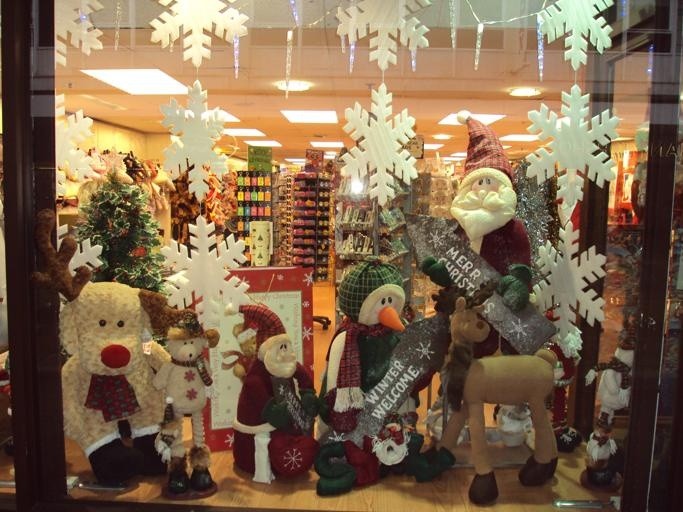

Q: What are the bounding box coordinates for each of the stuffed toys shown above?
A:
[545,307,583,453]
[316,255,441,495]
[33,209,198,484]
[580,313,636,492]
[438,297,559,504]
[153,313,213,494]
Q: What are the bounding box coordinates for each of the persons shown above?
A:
[418,110,532,446]
[232,305,319,484]
